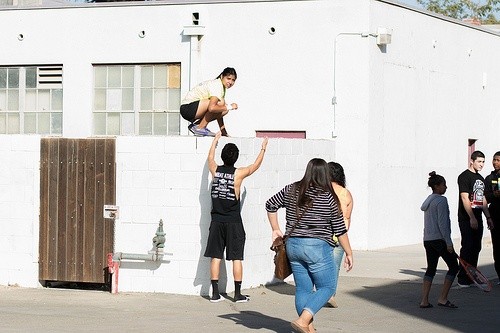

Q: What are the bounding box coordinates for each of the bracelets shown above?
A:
[487,217,491,220]
[261,149,266,152]
[226,104,232,111]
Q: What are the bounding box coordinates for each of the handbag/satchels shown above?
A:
[274,238,293,280]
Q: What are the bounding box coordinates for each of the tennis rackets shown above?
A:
[454,252,490,291]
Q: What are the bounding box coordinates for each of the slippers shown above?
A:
[236,295,251,303]
[419,303,433,308]
[437,300,458,309]
[210,294,226,302]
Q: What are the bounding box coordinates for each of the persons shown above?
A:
[420,171,459,309]
[204,132,268,303]
[180,67,239,137]
[485,151,500,286]
[321,162,353,309]
[266,158,353,333]
[457,151,494,287]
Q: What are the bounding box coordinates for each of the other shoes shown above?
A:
[471,280,482,286]
[327,298,337,307]
[291,321,317,333]
[194,129,215,136]
[457,279,471,287]
[188,123,199,133]
